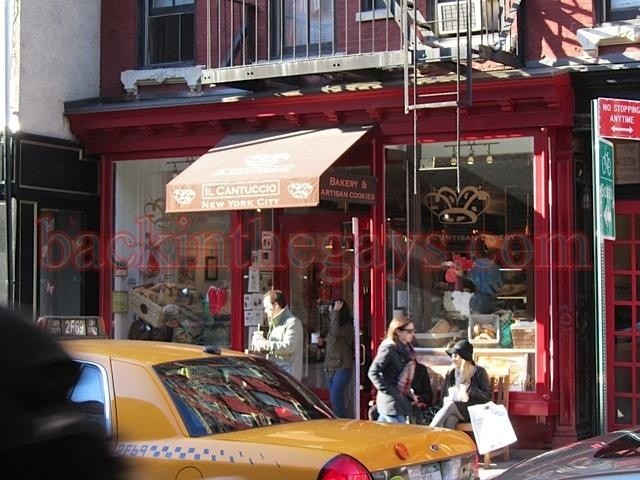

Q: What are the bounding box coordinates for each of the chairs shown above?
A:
[428,372,444,405]
[456,373,510,467]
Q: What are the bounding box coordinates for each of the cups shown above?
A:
[311,334,320,343]
[253,331,264,341]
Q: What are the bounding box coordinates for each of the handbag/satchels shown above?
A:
[468,401,518,456]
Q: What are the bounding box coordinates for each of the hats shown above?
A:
[446,339,474,362]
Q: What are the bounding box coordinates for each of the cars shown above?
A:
[35,316,480,480]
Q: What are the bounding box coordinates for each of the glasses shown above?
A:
[401,327,416,334]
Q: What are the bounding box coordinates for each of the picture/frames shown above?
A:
[205,256,218,280]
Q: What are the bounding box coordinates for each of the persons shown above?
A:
[368,315,418,425]
[467,245,502,297]
[318,299,354,419]
[411,363,433,425]
[430,340,492,429]
[251,290,303,381]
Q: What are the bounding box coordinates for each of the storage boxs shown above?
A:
[132,283,167,329]
[511,321,535,349]
[468,314,500,348]
[415,332,458,348]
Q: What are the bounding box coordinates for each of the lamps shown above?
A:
[167,157,196,179]
[445,140,499,166]
